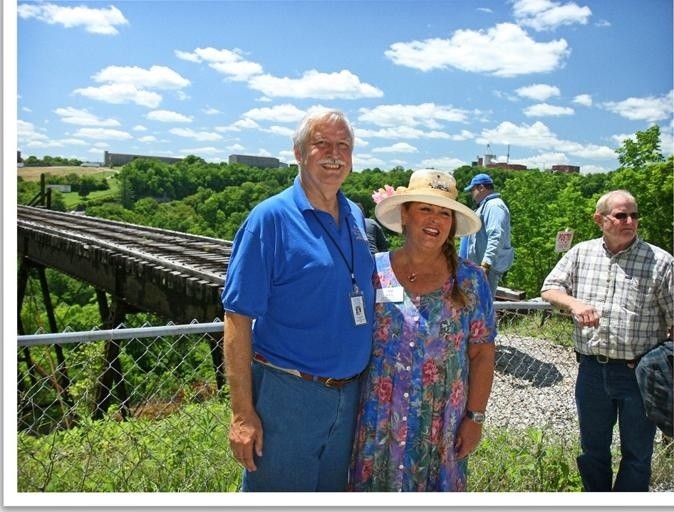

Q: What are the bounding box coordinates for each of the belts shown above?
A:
[254,354,360,388]
[576,353,636,368]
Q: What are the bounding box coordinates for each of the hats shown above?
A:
[375,169,481,236]
[465,173,492,190]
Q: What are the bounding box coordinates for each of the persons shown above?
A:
[345,168,500,491]
[457,172,515,302]
[217,104,376,492]
[540,188,674,491]
[356,201,390,254]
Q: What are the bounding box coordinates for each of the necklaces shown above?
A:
[401,247,443,284]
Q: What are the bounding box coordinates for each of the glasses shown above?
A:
[605,213,639,219]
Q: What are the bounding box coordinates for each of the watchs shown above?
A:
[465,410,488,426]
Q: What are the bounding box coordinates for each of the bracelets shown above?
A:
[480,262,491,269]
